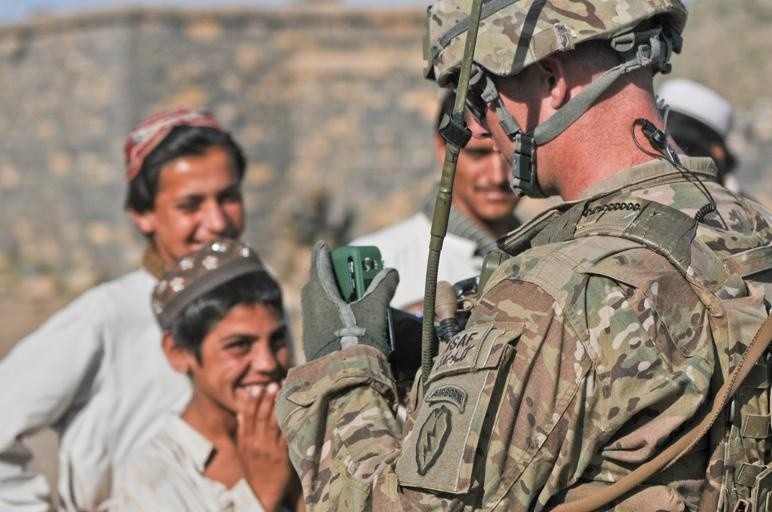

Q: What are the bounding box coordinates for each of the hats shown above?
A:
[151,238,266,331]
[656,78,733,137]
[123,105,233,186]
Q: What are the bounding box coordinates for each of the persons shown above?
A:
[656,76,741,195]
[350,88,526,323]
[0,109,289,512]
[272,0,772,512]
[112,238,305,512]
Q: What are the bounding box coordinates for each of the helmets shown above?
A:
[423,0,689,88]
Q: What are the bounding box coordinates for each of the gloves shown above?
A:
[300,240,400,362]
